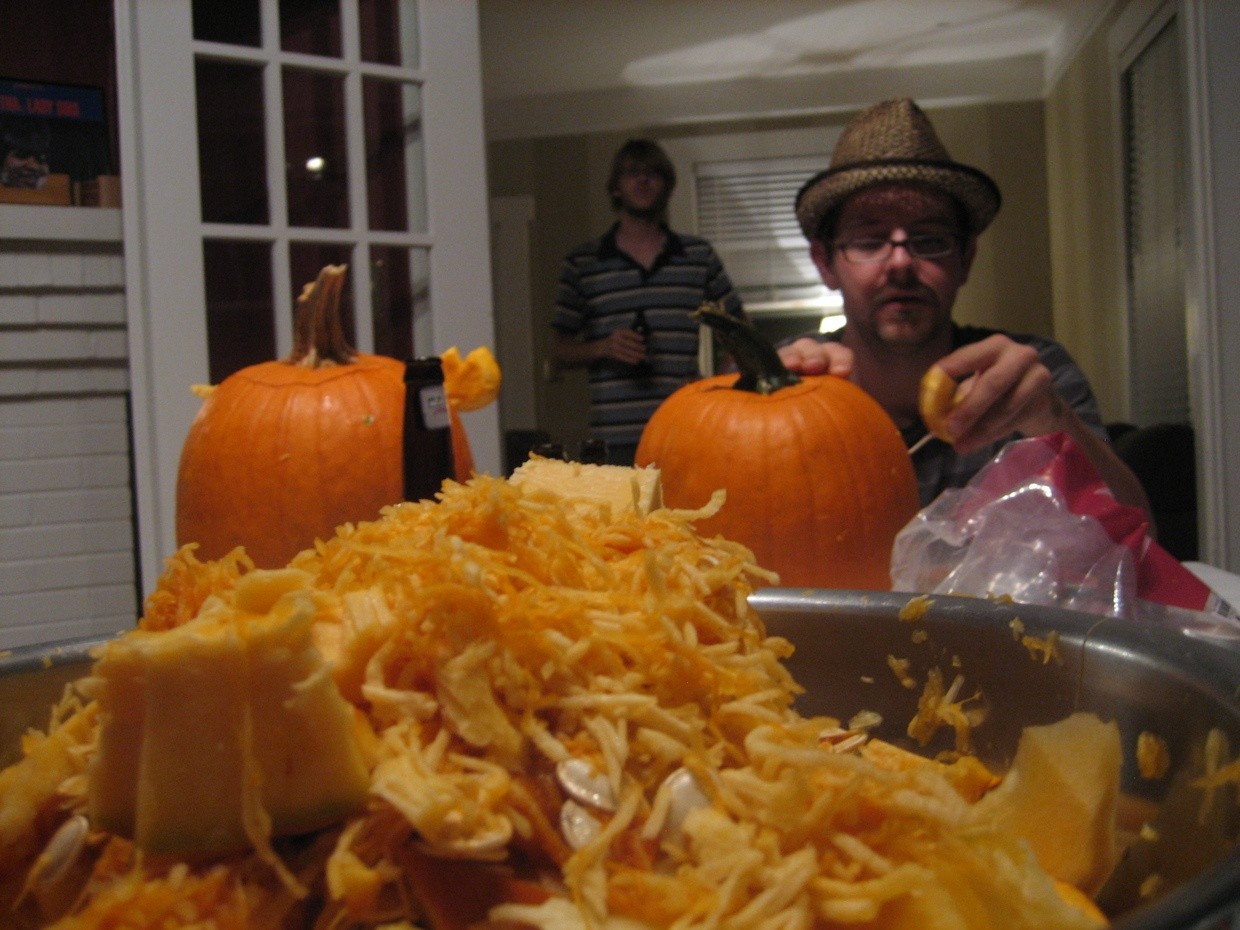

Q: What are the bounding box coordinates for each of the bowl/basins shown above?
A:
[0,586,1239,930]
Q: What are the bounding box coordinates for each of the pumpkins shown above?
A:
[637,305,921,600]
[170,264,478,573]
[435,344,503,412]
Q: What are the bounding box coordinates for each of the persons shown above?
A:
[722,98,1157,544]
[546,139,753,467]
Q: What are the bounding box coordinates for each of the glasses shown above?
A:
[823,229,973,264]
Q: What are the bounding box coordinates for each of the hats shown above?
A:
[794,97,1004,244]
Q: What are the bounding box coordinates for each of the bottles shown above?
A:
[399,352,459,503]
[627,305,651,377]
